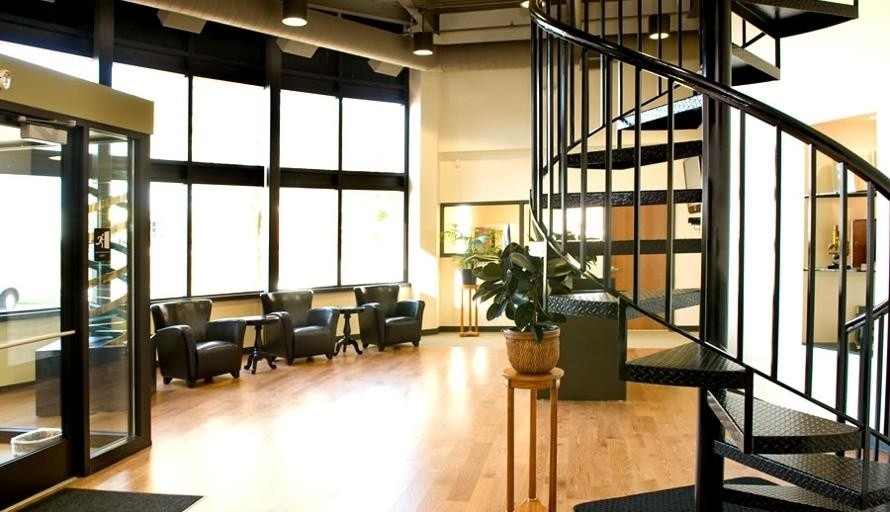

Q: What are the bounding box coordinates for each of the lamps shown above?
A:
[412,14,434,56]
[648,12,670,40]
[281,0,307,26]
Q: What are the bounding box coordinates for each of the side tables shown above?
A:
[334,305,367,356]
[235,315,281,374]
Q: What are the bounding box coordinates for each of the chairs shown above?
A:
[150,299,246,388]
[353,285,426,351]
[259,290,339,365]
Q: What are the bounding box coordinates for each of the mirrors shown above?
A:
[439,200,530,257]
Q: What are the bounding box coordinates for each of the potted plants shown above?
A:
[447,227,503,284]
[472,230,598,375]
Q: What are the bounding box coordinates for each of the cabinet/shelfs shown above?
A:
[500,366,567,512]
[801,108,877,355]
[459,284,481,337]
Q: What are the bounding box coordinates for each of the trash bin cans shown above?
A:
[10,427,63,459]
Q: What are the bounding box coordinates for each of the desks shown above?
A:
[36,329,157,418]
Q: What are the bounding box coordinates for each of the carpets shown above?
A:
[21,487,202,512]
